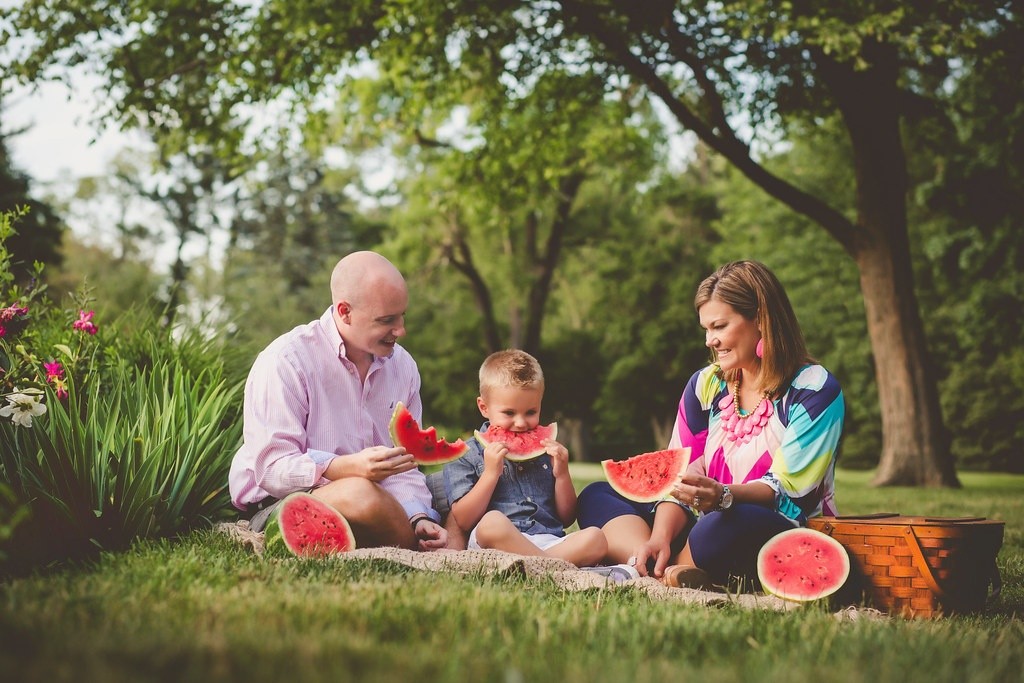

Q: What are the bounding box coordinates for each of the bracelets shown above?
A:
[411,516,439,529]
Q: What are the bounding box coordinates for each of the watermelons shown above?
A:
[601,447,691,502]
[474,422,557,462]
[264,492,356,558]
[757,528,850,601]
[388,401,470,465]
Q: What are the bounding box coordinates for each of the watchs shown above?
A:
[712,483,735,512]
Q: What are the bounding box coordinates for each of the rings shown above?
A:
[629,557,637,568]
[694,496,701,507]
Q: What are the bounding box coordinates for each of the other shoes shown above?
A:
[580,564,640,583]
[661,564,714,592]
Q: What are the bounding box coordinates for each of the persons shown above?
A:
[577,259,846,594]
[442,349,641,581]
[228,250,468,551]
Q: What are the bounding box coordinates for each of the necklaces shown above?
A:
[733,368,770,419]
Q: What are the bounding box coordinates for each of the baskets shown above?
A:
[806,513,1006,621]
